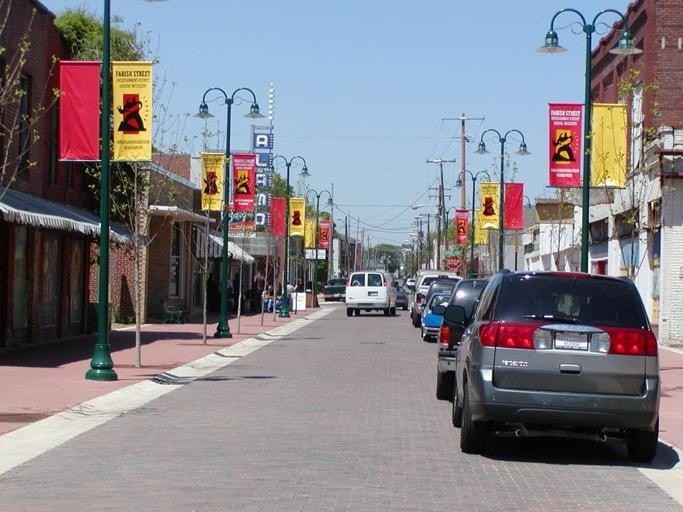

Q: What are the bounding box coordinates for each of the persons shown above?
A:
[253,270,265,305]
[263,285,273,313]
[233,273,245,315]
[286,282,298,306]
[206,274,217,311]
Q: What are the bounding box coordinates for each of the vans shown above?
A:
[429,277,485,403]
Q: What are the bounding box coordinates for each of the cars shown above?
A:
[325,267,472,343]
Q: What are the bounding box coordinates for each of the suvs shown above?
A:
[436,264,663,463]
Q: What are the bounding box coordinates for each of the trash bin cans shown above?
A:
[306,289,316,307]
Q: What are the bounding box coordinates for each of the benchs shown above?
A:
[160,298,190,323]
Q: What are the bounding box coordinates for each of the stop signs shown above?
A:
[445,254,462,270]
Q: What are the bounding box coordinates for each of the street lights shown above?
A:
[301,185,335,308]
[514,194,531,273]
[472,128,532,270]
[191,82,270,343]
[452,167,494,276]
[264,151,314,321]
[401,202,457,269]
[335,216,347,269]
[532,4,647,272]
